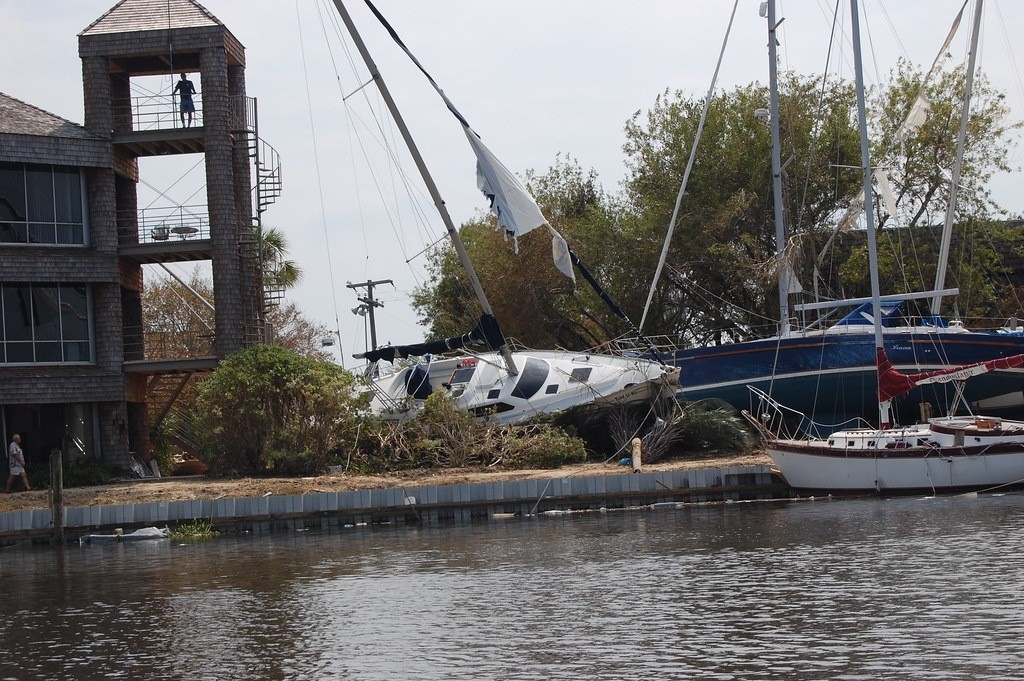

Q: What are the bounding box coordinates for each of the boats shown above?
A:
[77,525,175,544]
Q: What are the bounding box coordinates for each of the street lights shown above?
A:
[350,303,370,368]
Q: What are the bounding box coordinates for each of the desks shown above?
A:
[171,227,198,241]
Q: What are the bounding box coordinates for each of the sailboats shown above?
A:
[741,1,1024,497]
[602,11,1024,442]
[299,0,685,447]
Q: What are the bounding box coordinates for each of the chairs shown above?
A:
[151,227,170,243]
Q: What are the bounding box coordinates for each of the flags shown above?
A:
[433,81,576,283]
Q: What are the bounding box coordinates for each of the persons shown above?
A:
[4,434,38,494]
[172,72,196,128]
